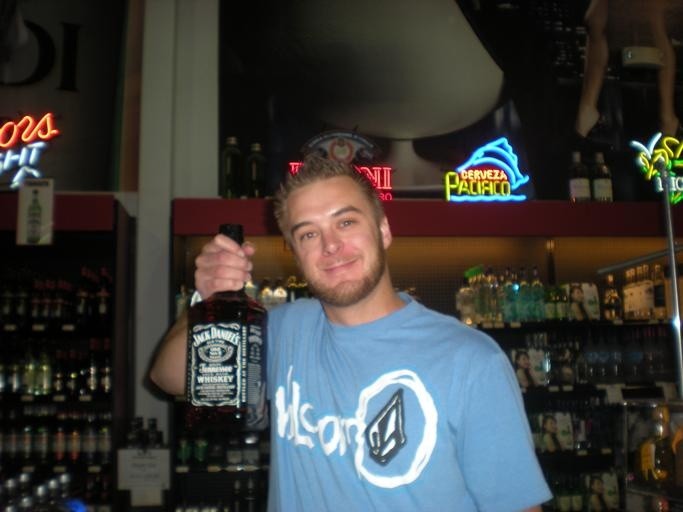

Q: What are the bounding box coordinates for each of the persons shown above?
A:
[150,153,554,512]
[554,0,683,171]
[540,413,564,451]
[569,285,594,321]
[586,476,610,512]
[513,350,543,391]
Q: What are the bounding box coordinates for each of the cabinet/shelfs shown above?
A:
[481,322,683,512]
[0,191,135,512]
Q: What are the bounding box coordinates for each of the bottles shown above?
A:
[592,148,614,203]
[184,223,270,433]
[457,261,682,383]
[174,433,262,468]
[568,149,591,202]
[0,282,110,512]
[125,415,164,450]
[541,473,586,512]
